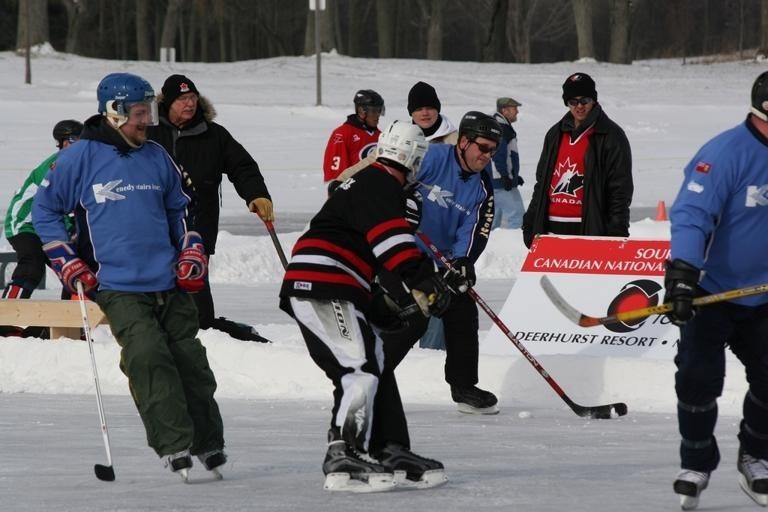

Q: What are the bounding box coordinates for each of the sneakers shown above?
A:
[373,443,446,473]
[737,444,768,495]
[322,428,392,476]
[195,451,229,469]
[673,468,711,494]
[160,450,194,471]
[452,380,498,408]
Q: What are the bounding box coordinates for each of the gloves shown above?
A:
[75,269,98,293]
[247,199,275,221]
[175,261,206,291]
[662,258,700,327]
[415,272,452,316]
[450,258,477,295]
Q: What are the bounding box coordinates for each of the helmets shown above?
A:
[97,73,155,114]
[375,120,429,171]
[53,119,84,140]
[458,111,502,144]
[354,90,384,107]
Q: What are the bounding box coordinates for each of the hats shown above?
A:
[751,71,767,114]
[161,74,199,107]
[408,81,442,114]
[496,97,522,108]
[563,72,598,101]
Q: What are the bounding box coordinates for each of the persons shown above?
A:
[32,73,228,484]
[407,81,458,146]
[1,120,89,303]
[523,72,632,249]
[663,71,768,508]
[279,120,453,493]
[393,111,500,413]
[490,97,524,228]
[324,89,385,181]
[144,75,273,327]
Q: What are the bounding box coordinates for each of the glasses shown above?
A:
[470,141,498,157]
[568,96,594,107]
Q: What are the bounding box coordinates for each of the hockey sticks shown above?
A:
[539,274,768,328]
[77,280,116,483]
[418,232,627,420]
